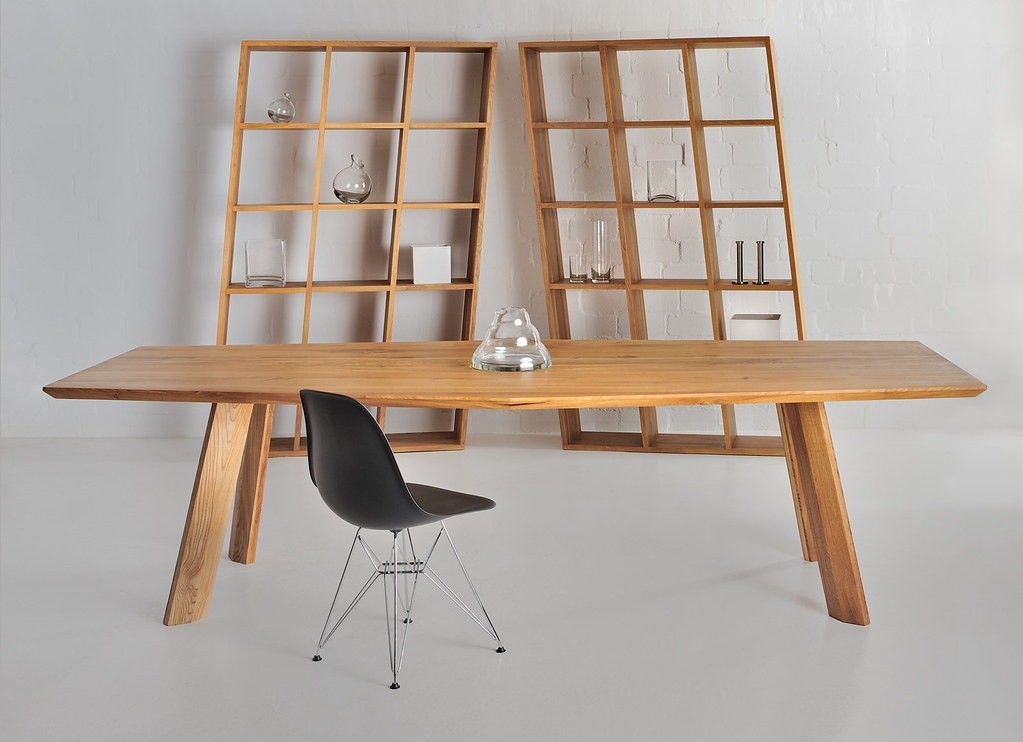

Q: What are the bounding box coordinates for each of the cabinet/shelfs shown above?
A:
[518,36,806,455]
[216,39,497,459]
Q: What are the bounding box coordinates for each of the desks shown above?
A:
[41,340,988,627]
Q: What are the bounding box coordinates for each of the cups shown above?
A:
[245,237,287,286]
[569,252,586,283]
[591,221,613,284]
[647,159,677,201]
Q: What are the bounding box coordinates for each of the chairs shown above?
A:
[299,390,505,690]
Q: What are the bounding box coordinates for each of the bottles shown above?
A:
[333,154,372,203]
[267,92,295,124]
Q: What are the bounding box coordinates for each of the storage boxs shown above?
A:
[409,245,451,284]
[729,314,780,341]
